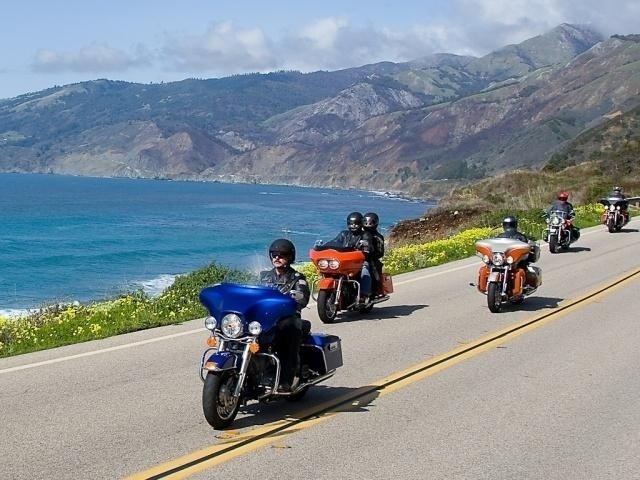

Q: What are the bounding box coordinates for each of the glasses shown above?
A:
[271,252,287,259]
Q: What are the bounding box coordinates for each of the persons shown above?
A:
[364,212,384,298]
[495,215,528,276]
[257,239,310,392]
[610,187,625,199]
[314,212,373,303]
[544,191,575,238]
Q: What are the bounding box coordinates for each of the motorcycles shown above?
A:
[594,195,633,233]
[467,227,547,313]
[540,202,581,255]
[307,240,395,322]
[196,250,346,428]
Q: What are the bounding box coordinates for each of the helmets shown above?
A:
[362,212,380,232]
[268,238,296,264]
[502,216,517,235]
[556,191,569,201]
[613,186,622,193]
[346,211,364,233]
